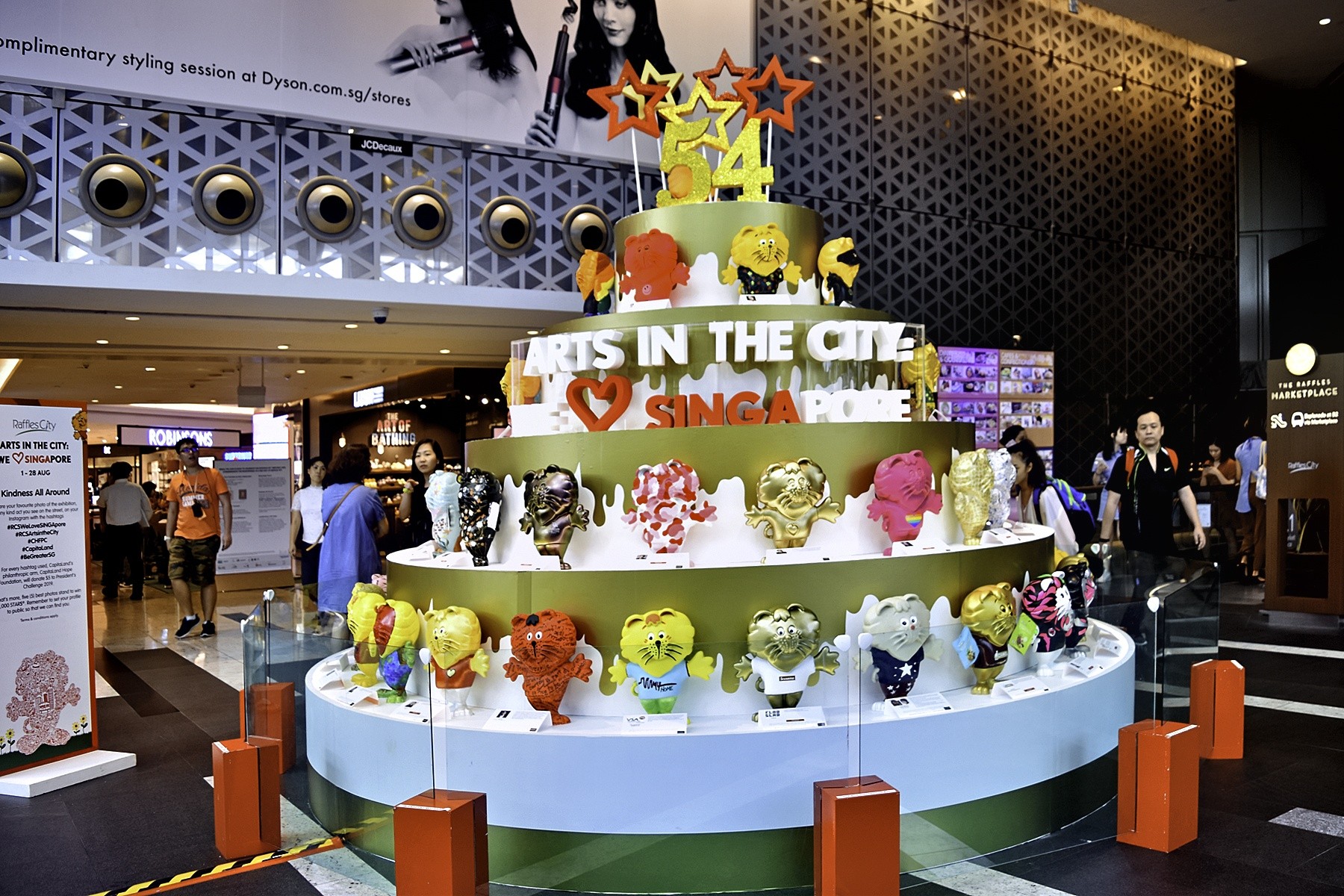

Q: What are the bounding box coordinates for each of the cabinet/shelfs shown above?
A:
[368,468,417,506]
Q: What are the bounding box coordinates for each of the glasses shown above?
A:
[180,447,199,453]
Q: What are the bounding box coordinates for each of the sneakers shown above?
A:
[200,620,215,638]
[175,613,201,639]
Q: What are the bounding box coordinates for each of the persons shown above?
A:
[525,0,680,164]
[996,425,1079,556]
[318,443,389,656]
[1200,414,1266,585]
[382,0,542,144]
[1097,407,1205,661]
[97,461,170,600]
[399,439,444,547]
[164,437,232,639]
[1092,420,1135,583]
[288,455,331,636]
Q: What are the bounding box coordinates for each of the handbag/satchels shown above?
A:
[1255,441,1267,499]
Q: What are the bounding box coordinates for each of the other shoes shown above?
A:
[1152,649,1171,660]
[1120,625,1147,646]
[311,625,331,636]
[103,592,118,601]
[130,594,142,601]
[1234,562,1266,585]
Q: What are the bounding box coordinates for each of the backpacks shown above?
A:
[1032,478,1096,553]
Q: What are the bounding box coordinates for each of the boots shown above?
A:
[1095,554,1112,583]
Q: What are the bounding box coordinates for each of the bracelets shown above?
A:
[1099,538,1110,543]
[1095,471,1101,476]
[403,488,413,494]
[164,536,172,541]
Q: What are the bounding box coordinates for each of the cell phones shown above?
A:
[191,504,203,518]
[1201,463,1211,468]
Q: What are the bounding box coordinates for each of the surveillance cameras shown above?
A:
[371,306,389,324]
